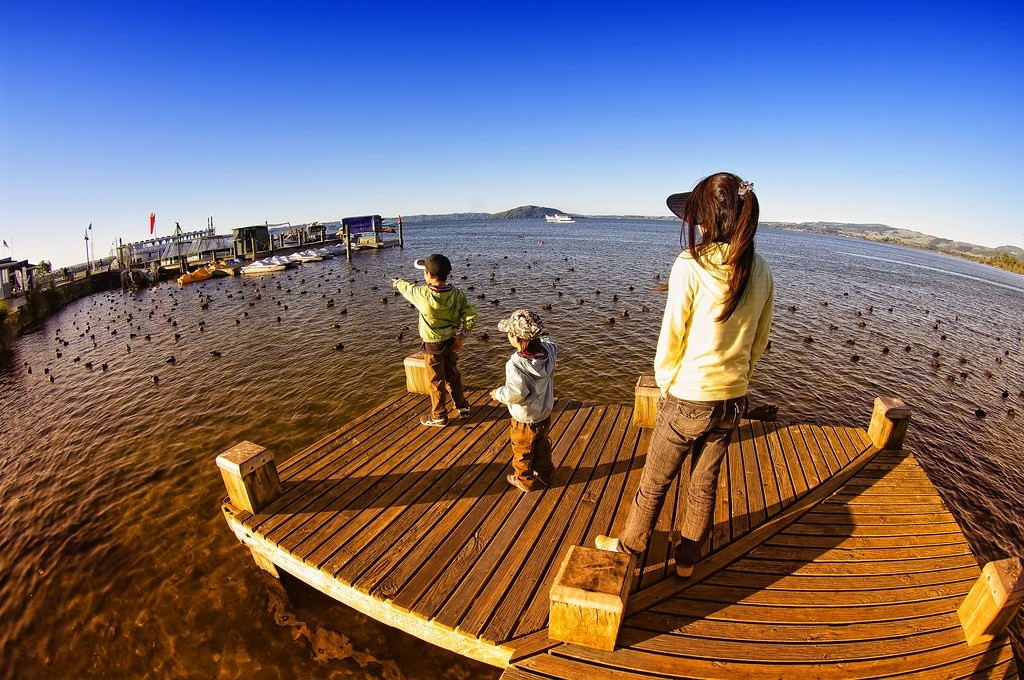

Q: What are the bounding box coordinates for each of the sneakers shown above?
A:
[507,474,533,492]
[420,412,448,427]
[533,471,553,485]
[458,406,470,419]
[595,535,641,567]
[672,540,694,577]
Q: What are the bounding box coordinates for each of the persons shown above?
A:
[390,253,479,427]
[490,309,558,492]
[60,267,71,280]
[27,273,35,289]
[595,171,775,578]
[98,259,103,267]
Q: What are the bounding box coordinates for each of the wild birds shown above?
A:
[20,228,1023,426]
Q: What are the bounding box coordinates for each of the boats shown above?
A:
[176,243,360,285]
[278,215,397,243]
[117,211,235,269]
[545,214,575,224]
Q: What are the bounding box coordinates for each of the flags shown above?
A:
[3,241,9,248]
[88,224,92,230]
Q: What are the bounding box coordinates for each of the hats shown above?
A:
[414,254,452,276]
[667,192,702,225]
[497,309,544,340]
